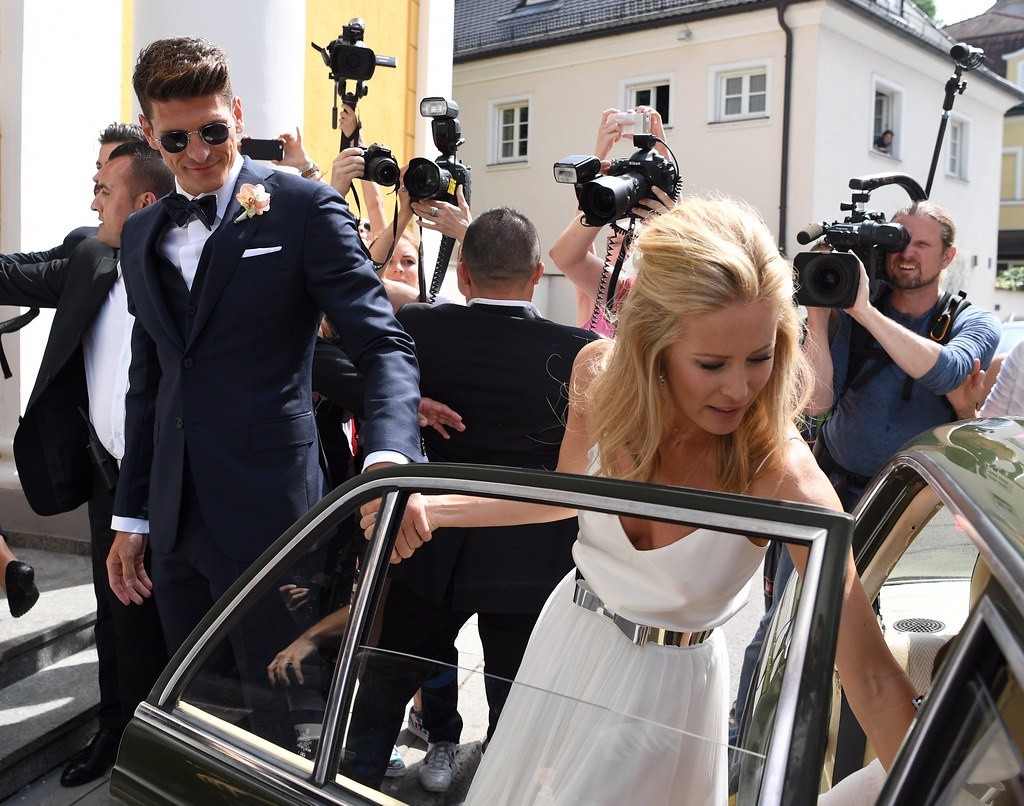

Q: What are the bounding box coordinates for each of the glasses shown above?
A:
[356,220,372,233]
[150,99,233,154]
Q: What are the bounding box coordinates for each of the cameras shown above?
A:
[552,134,676,227]
[353,143,400,187]
[403,97,472,209]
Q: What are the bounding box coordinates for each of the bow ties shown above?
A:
[161,193,217,231]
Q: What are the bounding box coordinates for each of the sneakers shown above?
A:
[384,743,406,777]
[418,740,458,792]
[407,707,462,752]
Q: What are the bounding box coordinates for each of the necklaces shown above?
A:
[681,436,712,486]
[668,421,683,447]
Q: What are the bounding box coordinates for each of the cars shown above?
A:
[109,413,1024,806]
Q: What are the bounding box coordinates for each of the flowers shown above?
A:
[233,182,271,223]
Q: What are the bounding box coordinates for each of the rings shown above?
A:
[430,207,438,217]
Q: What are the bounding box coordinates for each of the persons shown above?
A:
[104,35,430,757]
[358,195,924,806]
[0,105,1024,788]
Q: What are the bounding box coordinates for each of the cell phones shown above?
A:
[607,113,651,135]
[241,139,283,161]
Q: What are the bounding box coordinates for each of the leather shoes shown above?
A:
[5,561,39,618]
[59,725,125,788]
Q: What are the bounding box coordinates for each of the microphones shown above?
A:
[949,42,969,60]
[796,224,825,245]
